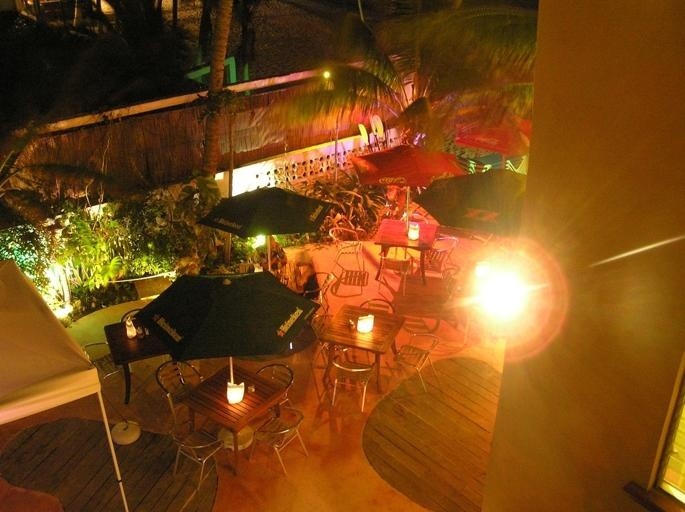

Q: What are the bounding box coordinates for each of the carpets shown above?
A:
[0,416,218,512]
[361,357,505,510]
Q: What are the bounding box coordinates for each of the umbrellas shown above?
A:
[194,187,334,273]
[409,169,526,247]
[350,145,469,260]
[132,269,320,436]
[456,116,530,172]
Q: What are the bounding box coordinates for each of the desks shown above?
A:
[374,217,439,285]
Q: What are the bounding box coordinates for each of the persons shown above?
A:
[174,247,200,276]
[293,262,319,299]
[197,238,237,275]
[258,235,287,272]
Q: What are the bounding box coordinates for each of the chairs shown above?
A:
[82,228,459,490]
